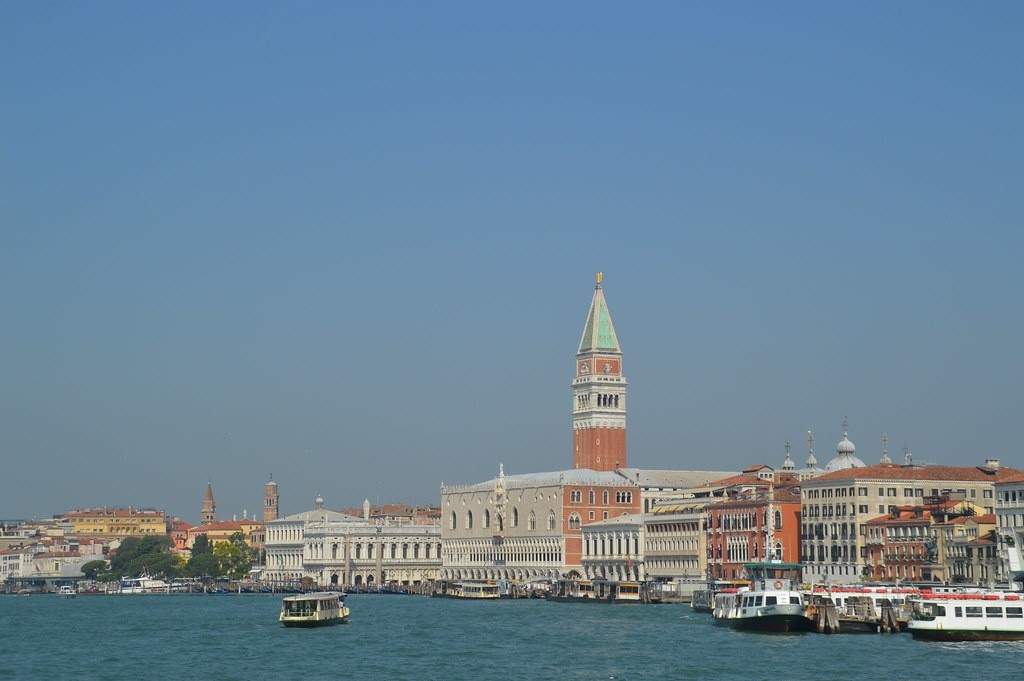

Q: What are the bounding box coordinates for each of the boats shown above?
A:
[446,578,497,595]
[59,585,77,595]
[278,591,350,628]
[449,582,501,598]
[18,589,31,596]
[906,592,1024,641]
[649,581,682,603]
[713,562,808,631]
[690,589,715,610]
[118,566,171,594]
[543,581,647,604]
[811,587,934,634]
